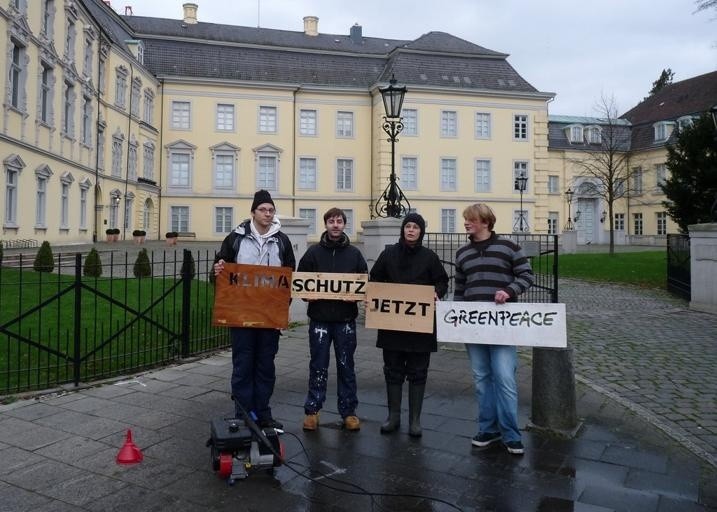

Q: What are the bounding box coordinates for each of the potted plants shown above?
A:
[106,228,120,242]
[165,232,177,246]
[133,230,146,245]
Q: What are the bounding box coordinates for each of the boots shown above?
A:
[381,386,402,431]
[409,384,425,436]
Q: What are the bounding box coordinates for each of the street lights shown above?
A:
[513,174,530,232]
[564,186,575,231]
[375,74,411,219]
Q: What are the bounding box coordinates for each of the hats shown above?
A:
[251,190,275,211]
[401,213,425,238]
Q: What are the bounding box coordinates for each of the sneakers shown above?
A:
[472,431,502,447]
[270,421,284,429]
[504,440,525,454]
[304,414,317,430]
[343,416,360,430]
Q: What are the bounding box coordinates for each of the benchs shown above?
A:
[176,232,196,237]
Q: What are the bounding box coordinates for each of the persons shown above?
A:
[210,190,296,429]
[296,207,368,432]
[453,204,534,454]
[364,213,449,436]
